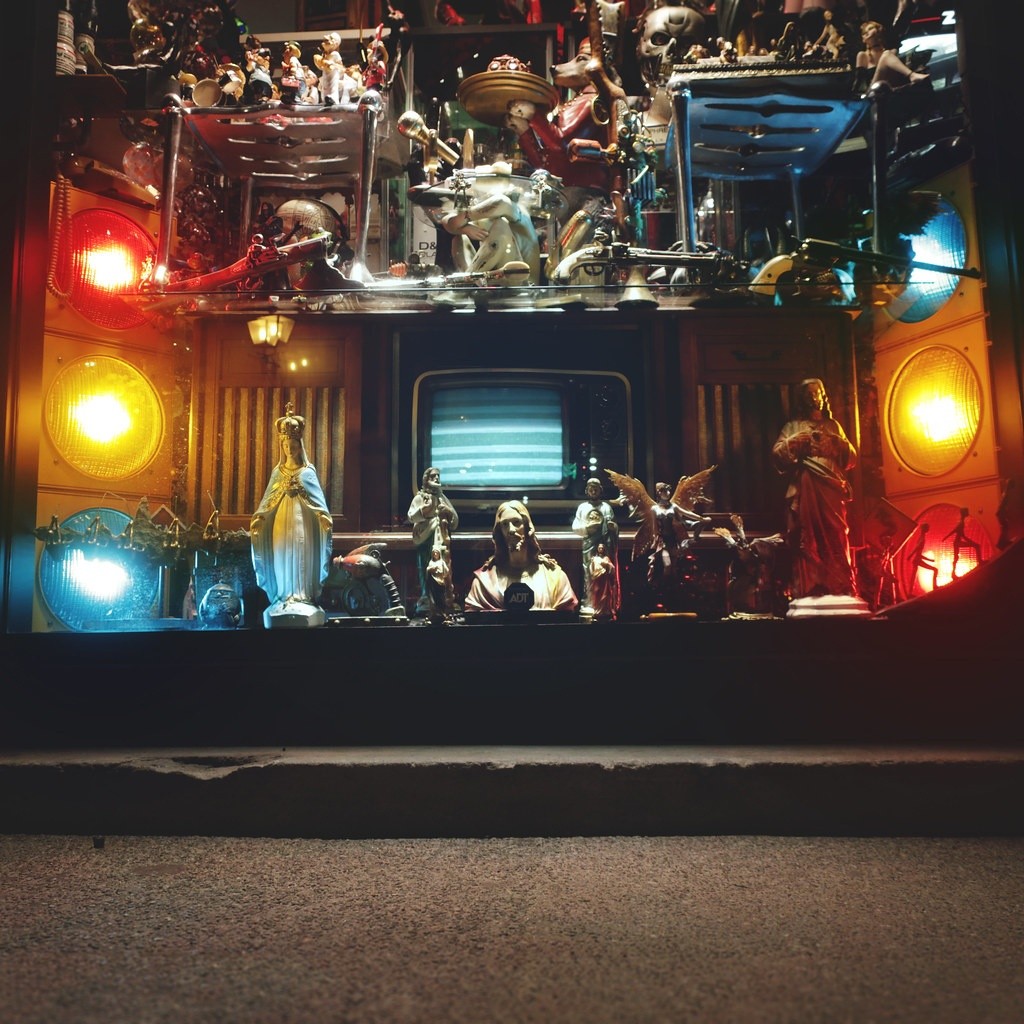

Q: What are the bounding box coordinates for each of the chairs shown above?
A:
[664,67,868,276]
[164,42,414,284]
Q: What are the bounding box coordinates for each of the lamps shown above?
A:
[248,313,294,375]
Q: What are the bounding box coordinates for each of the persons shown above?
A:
[362,39,388,104]
[588,542,616,613]
[906,523,941,600]
[814,9,842,61]
[774,379,861,599]
[426,545,451,611]
[571,478,618,606]
[464,500,578,610]
[581,508,610,608]
[253,201,291,289]
[319,32,342,104]
[251,403,335,604]
[873,534,896,609]
[942,507,987,581]
[775,21,806,60]
[407,467,458,605]
[301,69,318,105]
[856,20,929,90]
[239,36,273,104]
[604,464,719,583]
[281,39,308,105]
[995,478,1018,550]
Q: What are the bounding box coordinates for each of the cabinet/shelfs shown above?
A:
[160,18,866,310]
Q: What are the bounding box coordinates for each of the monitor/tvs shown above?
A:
[409,369,636,519]
